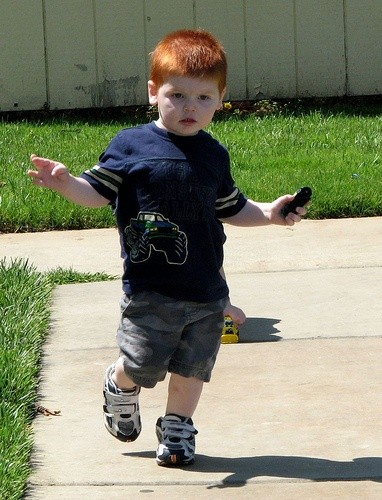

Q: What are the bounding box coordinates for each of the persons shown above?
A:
[27,28,312,467]
[219,266,246,324]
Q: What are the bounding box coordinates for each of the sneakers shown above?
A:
[102,363,141,442]
[155,414,197,466]
